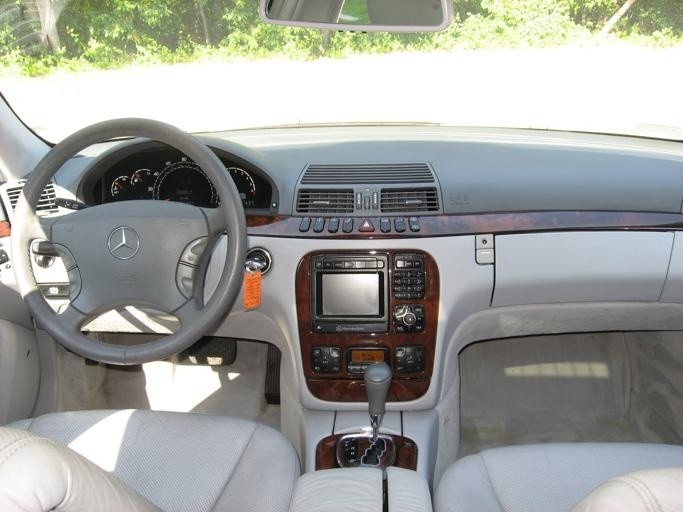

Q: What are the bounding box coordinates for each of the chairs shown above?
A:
[3,410,303,511]
[434,438,683,512]
